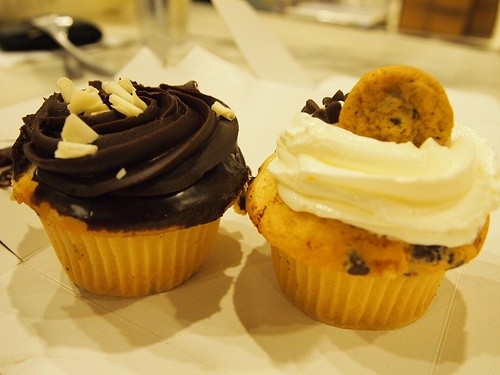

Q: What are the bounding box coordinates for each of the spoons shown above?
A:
[28,12,117,76]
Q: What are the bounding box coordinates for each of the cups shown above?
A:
[129,2,192,71]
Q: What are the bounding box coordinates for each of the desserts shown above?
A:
[247,65,491,330]
[0,73,252,297]
